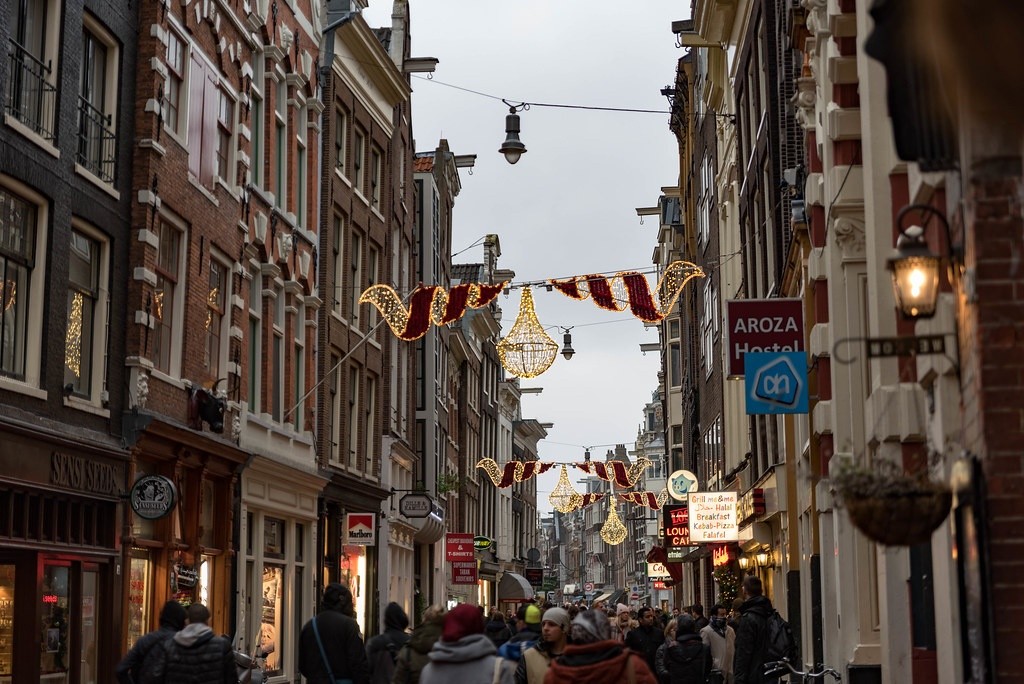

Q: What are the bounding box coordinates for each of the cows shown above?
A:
[191,375,241,434]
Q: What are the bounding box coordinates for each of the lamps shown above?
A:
[885,203,955,321]
[737,552,755,576]
[756,546,776,571]
[498,100,590,465]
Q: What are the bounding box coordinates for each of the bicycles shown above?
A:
[763,657,841,684]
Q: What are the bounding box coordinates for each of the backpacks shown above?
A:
[746,608,798,680]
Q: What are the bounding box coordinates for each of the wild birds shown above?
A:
[64,383,74,397]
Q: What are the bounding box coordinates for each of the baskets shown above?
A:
[849,488,951,545]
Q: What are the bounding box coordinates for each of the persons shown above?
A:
[153,603,238,684]
[115,601,189,684]
[733,575,773,684]
[364,598,745,684]
[299,583,369,684]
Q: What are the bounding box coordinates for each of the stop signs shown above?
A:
[631,594,639,600]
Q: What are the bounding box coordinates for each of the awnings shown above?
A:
[498,573,534,603]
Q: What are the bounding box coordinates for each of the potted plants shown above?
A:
[834,453,952,550]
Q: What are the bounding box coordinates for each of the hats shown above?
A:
[524,606,542,623]
[616,603,630,616]
[732,598,743,610]
[542,607,570,631]
[442,603,483,641]
